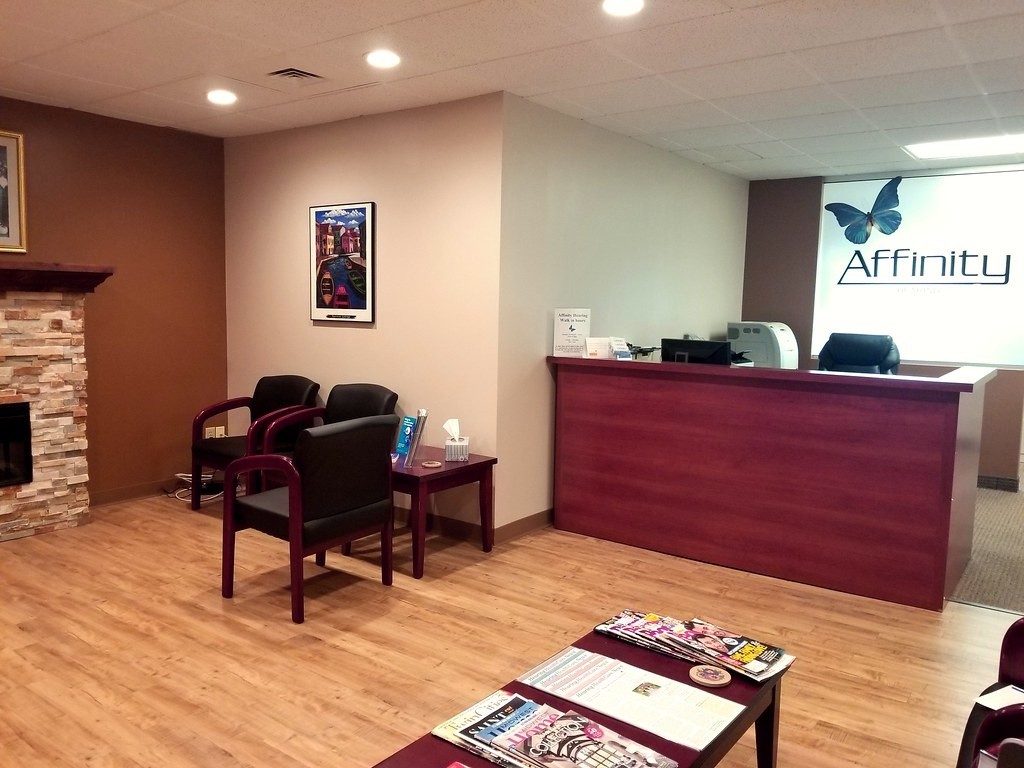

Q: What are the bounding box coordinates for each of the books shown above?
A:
[390,408,429,466]
[515,646,746,752]
[430,690,678,768]
[592,609,796,682]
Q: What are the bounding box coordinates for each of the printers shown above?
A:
[727,321,799,370]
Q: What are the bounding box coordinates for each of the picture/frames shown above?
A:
[308,202,376,324]
[0,129,29,254]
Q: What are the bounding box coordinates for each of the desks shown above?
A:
[342,444,498,579]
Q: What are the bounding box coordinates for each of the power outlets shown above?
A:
[216,426,225,439]
[205,427,216,439]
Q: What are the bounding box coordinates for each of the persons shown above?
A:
[692,634,768,674]
[684,621,785,665]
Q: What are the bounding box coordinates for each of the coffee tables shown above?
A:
[373,610,795,768]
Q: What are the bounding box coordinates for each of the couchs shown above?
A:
[956,617,1024,768]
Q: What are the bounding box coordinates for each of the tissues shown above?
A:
[441,418,470,462]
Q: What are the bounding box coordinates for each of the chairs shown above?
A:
[260,383,399,492]
[192,375,320,510]
[818,332,901,374]
[221,414,400,624]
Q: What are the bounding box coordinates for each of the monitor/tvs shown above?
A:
[661,339,731,366]
